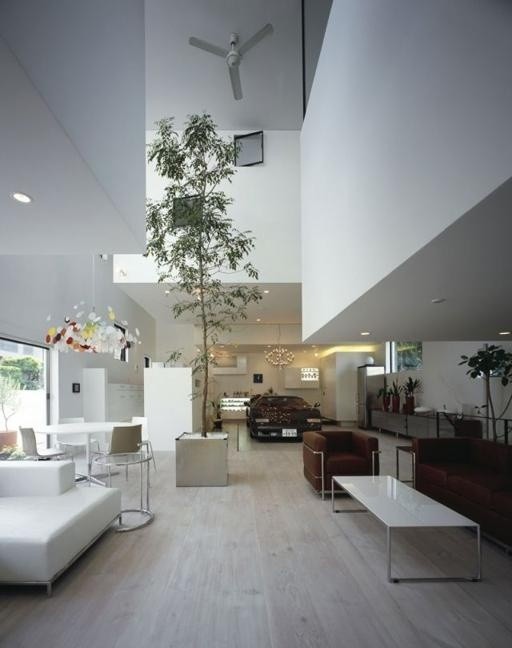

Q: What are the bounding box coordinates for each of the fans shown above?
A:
[189,23,274,100]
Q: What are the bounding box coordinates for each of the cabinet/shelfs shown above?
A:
[371,409,407,438]
[219,397,251,419]
[407,413,482,440]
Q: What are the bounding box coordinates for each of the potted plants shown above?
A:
[0,365,23,452]
[142,111,262,487]
[376,377,421,415]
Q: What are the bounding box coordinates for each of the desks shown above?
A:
[91,452,154,532]
[396,446,415,482]
[35,422,133,486]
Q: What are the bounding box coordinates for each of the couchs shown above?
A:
[0,461,122,598]
[411,437,512,556]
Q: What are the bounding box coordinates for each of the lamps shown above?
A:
[265,324,295,370]
[45,256,141,353]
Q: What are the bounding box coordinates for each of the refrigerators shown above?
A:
[357,364,385,429]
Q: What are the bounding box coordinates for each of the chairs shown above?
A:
[60,418,101,462]
[106,424,143,488]
[303,431,381,501]
[19,425,65,461]
[125,416,159,479]
